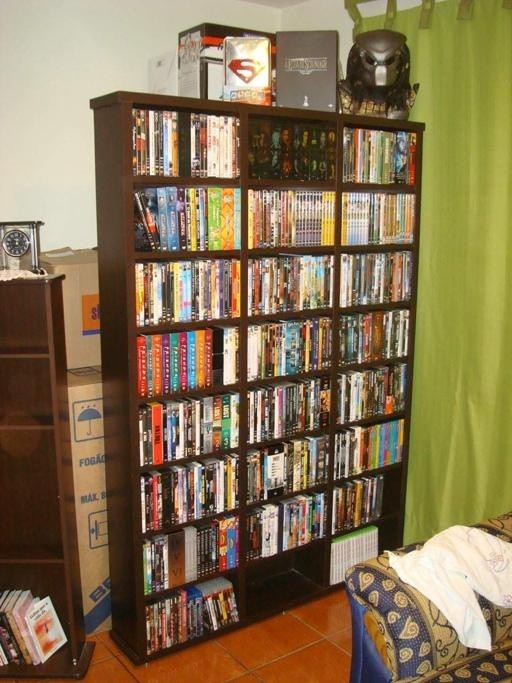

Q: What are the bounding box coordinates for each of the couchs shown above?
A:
[345,511,512,683]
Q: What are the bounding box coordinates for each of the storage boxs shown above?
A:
[39,246,101,371]
[68,364,113,638]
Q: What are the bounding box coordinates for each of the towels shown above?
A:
[387,525,512,652]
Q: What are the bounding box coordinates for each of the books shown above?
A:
[145,578,240,657]
[0,587,71,667]
[131,108,418,184]
[221,34,273,107]
[139,417,405,596]
[274,28,338,112]
[136,250,412,327]
[136,310,410,397]
[131,187,416,252]
[137,365,407,467]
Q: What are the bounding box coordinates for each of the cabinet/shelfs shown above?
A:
[1,274,95,679]
[91,90,425,665]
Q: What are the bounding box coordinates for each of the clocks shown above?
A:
[0,221,44,274]
